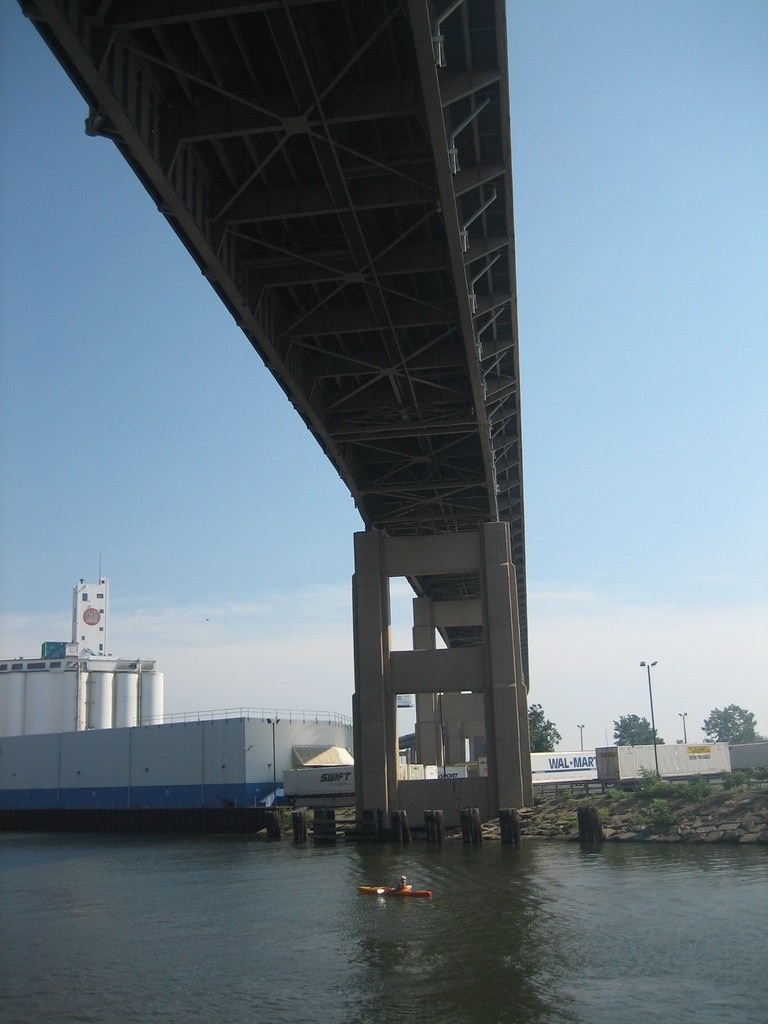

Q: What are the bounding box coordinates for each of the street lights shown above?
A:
[577,724,584,751]
[678,713,688,745]
[640,661,662,781]
[266,717,281,806]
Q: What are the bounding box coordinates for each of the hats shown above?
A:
[401,876,406,882]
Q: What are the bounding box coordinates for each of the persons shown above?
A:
[397,876,411,892]
